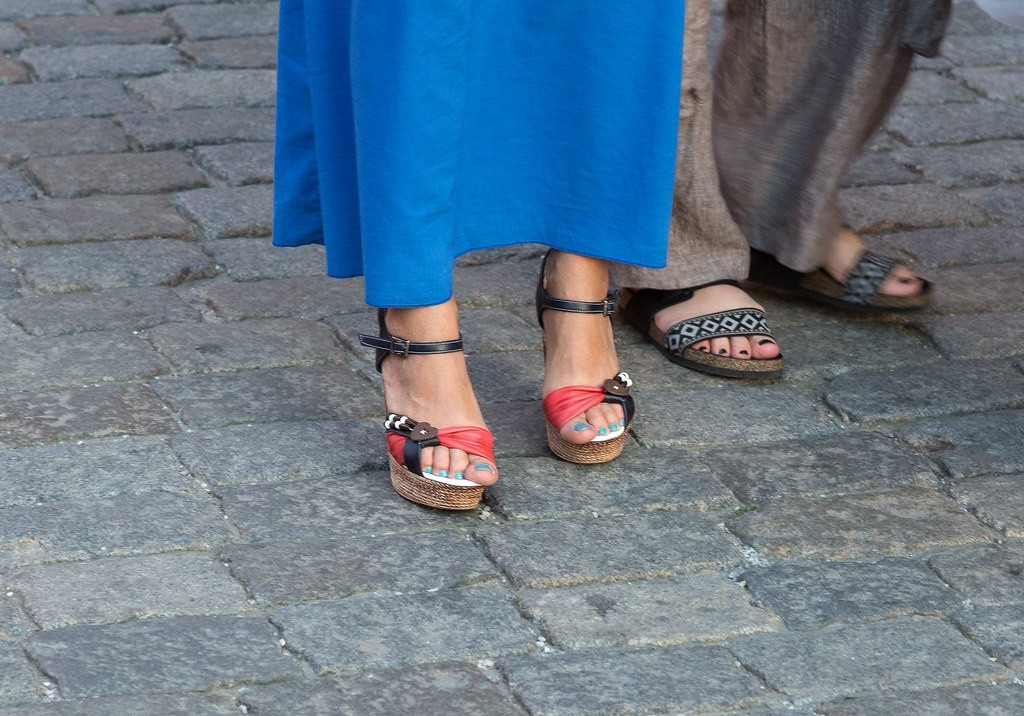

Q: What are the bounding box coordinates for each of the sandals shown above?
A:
[615,279,783,378]
[749,247,936,313]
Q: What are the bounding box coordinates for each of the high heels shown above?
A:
[358,307,496,509]
[536,247,635,464]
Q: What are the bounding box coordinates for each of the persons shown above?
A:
[271,0,686,511]
[609,0,953,378]
